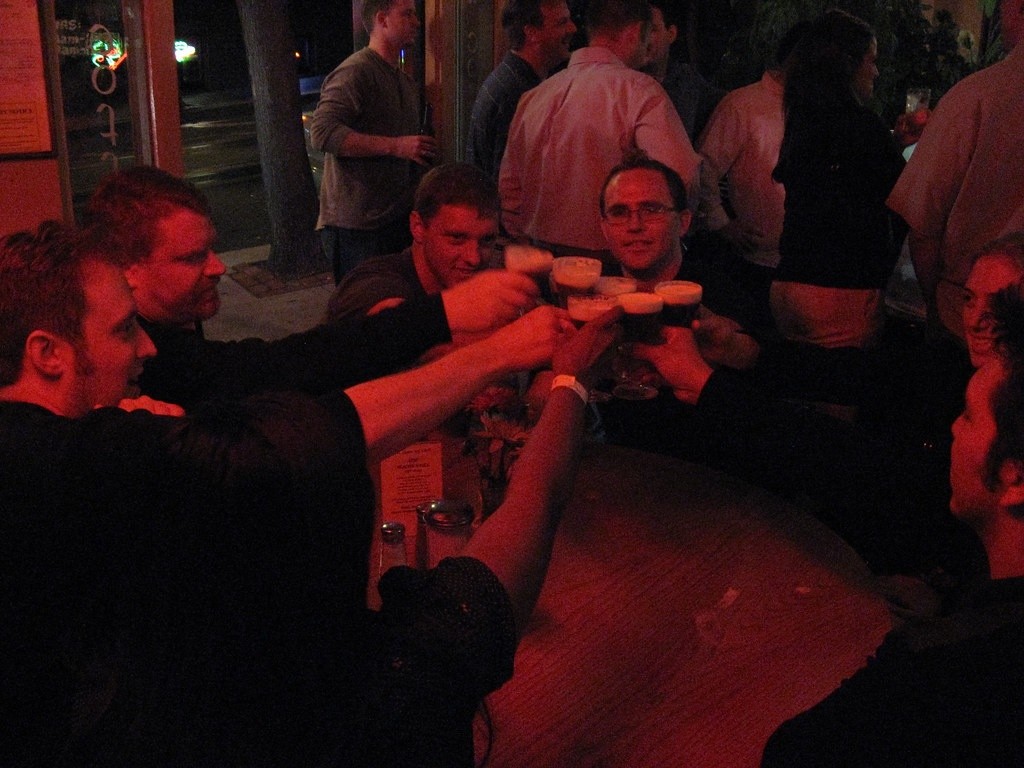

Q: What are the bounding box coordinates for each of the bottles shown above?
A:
[414,499,474,568]
[407,102,436,195]
[380,522,408,578]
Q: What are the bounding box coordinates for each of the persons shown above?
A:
[0,0,1024,768]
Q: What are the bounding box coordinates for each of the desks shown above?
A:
[369,422,891,768]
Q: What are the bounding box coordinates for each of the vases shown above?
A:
[471,472,504,529]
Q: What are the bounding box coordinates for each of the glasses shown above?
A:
[602,204,681,225]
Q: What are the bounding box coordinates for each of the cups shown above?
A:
[586,275,638,299]
[565,293,619,403]
[551,255,603,310]
[506,245,553,315]
[615,291,665,400]
[653,280,703,327]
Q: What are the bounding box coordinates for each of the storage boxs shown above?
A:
[376,440,444,537]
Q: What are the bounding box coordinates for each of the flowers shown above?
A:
[470,385,536,485]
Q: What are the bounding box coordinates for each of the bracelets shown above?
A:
[549,374,590,405]
